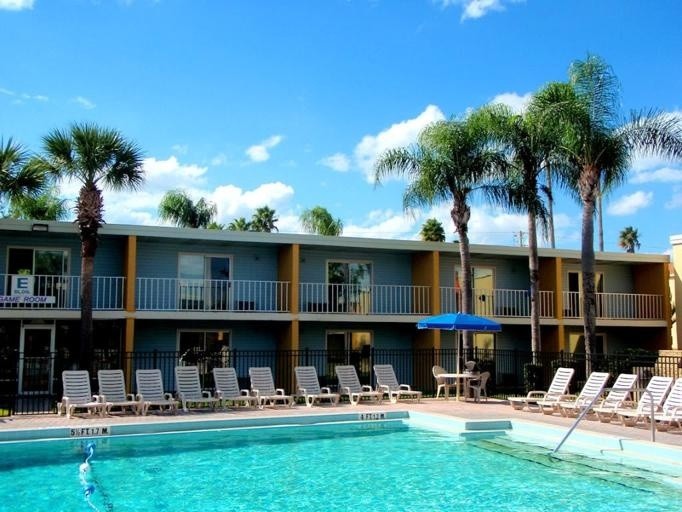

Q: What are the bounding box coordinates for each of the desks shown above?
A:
[438,373,482,401]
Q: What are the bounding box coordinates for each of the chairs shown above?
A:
[97,368,141,418]
[247,363,294,412]
[133,366,176,415]
[464,371,493,403]
[332,363,382,407]
[172,365,216,413]
[291,364,341,410]
[432,362,460,400]
[208,364,254,411]
[55,368,104,420]
[373,363,425,405]
[508,366,682,433]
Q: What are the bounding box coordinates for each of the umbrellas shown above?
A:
[416,312,502,373]
